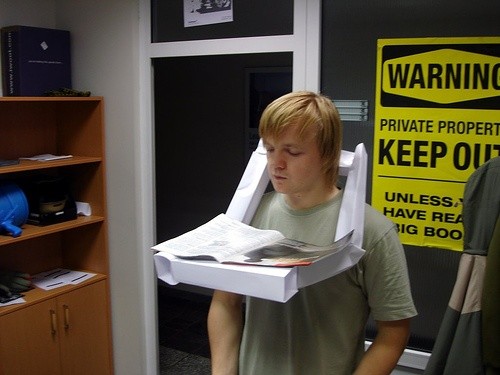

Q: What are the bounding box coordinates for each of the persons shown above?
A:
[207,91,419,375]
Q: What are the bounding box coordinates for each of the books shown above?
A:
[150,213,355,267]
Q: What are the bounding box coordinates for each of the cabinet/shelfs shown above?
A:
[0,96,115,375]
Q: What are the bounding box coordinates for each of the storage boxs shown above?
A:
[0,25,72,97]
